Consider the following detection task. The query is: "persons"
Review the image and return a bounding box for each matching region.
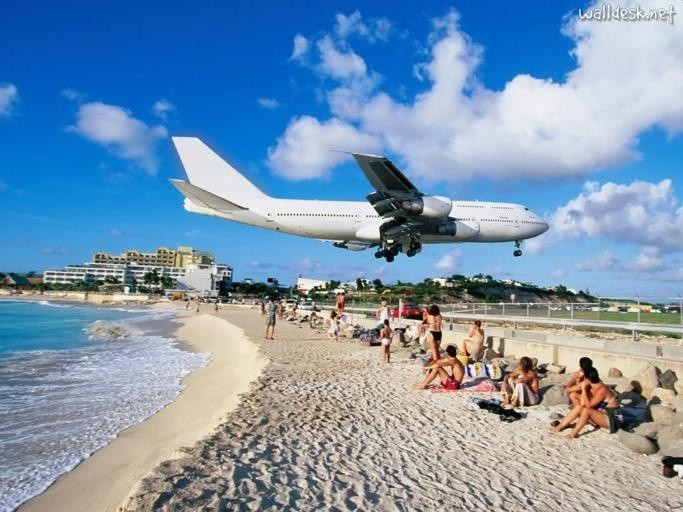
[276,302,283,319]
[563,356,593,408]
[287,307,296,317]
[263,296,276,339]
[375,297,388,325]
[299,314,309,322]
[409,344,465,391]
[259,300,265,315]
[378,318,392,363]
[498,356,540,408]
[420,304,443,361]
[460,319,482,337]
[326,310,340,339]
[550,366,626,439]
[310,312,317,326]
[335,291,344,313]
[333,292,340,304]
[458,327,484,357]
[415,307,432,339]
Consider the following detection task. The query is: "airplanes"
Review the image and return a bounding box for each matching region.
[171,135,549,264]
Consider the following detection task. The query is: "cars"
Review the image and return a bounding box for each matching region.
[280,298,316,310]
[392,303,423,319]
